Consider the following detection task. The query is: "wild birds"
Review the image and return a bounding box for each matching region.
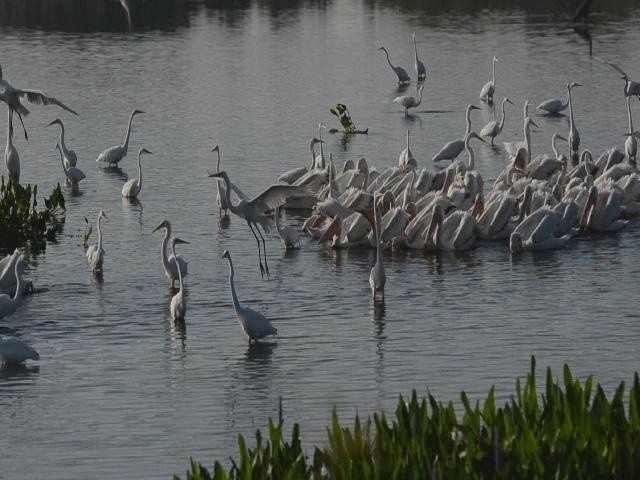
[4,104,154,196]
[375,32,430,85]
[394,86,429,118]
[149,218,192,320]
[0,247,41,365]
[619,71,640,99]
[210,98,640,298]
[474,54,502,109]
[536,76,583,151]
[85,210,108,272]
[219,250,278,346]
